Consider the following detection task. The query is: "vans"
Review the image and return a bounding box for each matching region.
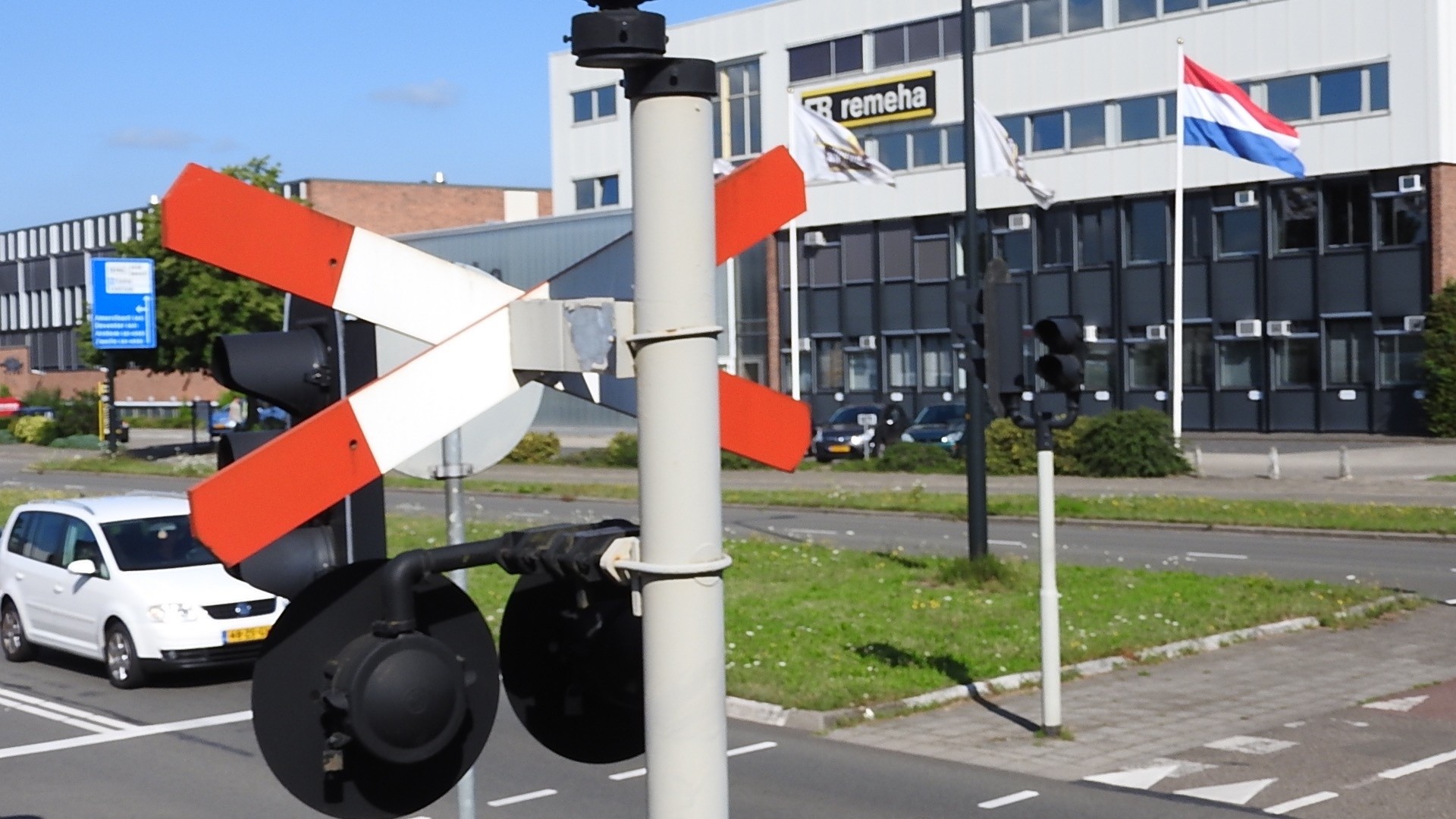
[0,487,291,690]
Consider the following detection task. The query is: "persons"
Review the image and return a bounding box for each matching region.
[228,395,242,424]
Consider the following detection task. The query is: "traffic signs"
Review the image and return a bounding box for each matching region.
[91,257,157,350]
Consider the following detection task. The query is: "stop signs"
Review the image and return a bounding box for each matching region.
[0,396,21,417]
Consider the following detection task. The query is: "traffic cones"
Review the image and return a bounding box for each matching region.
[1338,446,1352,480]
[1266,447,1280,479]
[1192,448,1205,477]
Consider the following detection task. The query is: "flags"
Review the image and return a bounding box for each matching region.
[1177,52,1306,180]
[972,98,1061,210]
[785,94,899,190]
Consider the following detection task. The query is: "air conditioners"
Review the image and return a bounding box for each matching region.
[1007,213,1031,231]
[1084,326,1099,341]
[1235,319,1262,340]
[804,232,826,249]
[1404,315,1426,332]
[859,335,876,350]
[1397,173,1421,194]
[1234,189,1254,206]
[799,337,811,352]
[1145,324,1167,341]
[1265,320,1291,335]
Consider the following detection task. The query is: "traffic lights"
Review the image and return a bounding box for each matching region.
[211,290,389,606]
[1033,314,1085,391]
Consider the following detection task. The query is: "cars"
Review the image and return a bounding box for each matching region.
[901,402,995,460]
[207,399,287,436]
[815,402,892,463]
